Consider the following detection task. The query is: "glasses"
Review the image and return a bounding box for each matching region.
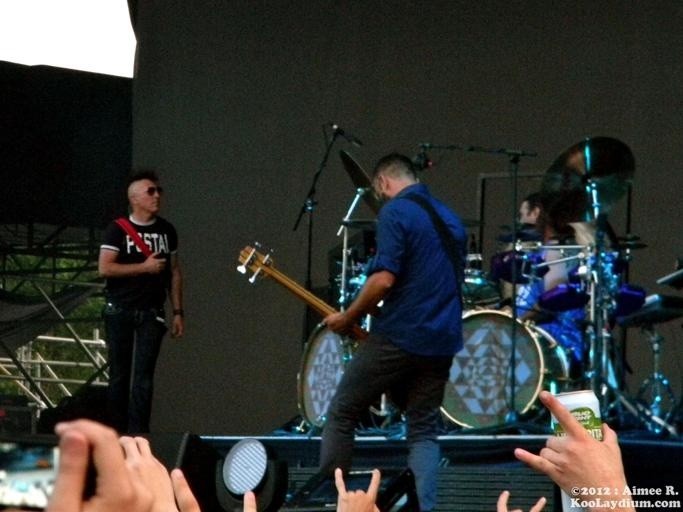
[136,186,164,198]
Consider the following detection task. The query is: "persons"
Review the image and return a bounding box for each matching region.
[99,178,184,435]
[502,192,578,320]
[315,152,468,509]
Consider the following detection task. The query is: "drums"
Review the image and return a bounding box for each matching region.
[460,252,501,306]
[296,321,365,430]
[540,135,636,223]
[439,309,566,429]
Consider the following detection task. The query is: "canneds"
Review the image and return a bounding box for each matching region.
[550,389,603,512]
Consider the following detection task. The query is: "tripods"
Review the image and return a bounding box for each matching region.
[514,180,683,442]
[278,190,406,436]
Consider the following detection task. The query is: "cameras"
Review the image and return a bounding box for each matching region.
[0,434,97,512]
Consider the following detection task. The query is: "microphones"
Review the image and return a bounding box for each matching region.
[328,119,362,147]
[411,154,438,172]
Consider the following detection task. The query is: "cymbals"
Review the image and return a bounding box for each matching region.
[497,223,539,243]
[340,149,383,214]
[489,251,548,284]
[337,218,375,230]
